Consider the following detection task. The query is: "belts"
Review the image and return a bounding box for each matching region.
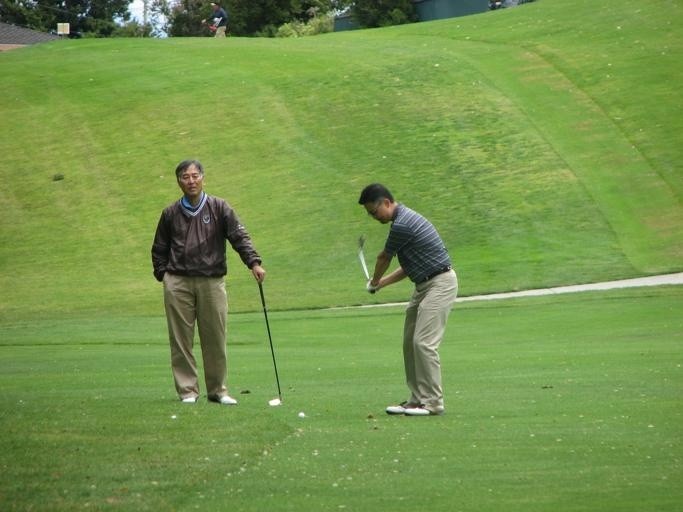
[417,265,451,284]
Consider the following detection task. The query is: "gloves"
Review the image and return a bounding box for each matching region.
[367,281,380,291]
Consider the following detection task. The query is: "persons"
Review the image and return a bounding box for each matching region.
[151,160,265,406]
[201,2,229,38]
[359,183,459,415]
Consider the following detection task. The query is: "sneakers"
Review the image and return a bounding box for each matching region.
[387,406,403,414]
[217,396,237,405]
[404,408,431,416]
[182,396,199,402]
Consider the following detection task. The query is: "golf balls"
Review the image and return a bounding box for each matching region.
[298,411,306,418]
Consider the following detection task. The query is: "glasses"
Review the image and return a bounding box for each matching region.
[367,201,382,216]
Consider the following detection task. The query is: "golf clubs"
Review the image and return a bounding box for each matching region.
[357,232,376,294]
[258,280,284,406]
